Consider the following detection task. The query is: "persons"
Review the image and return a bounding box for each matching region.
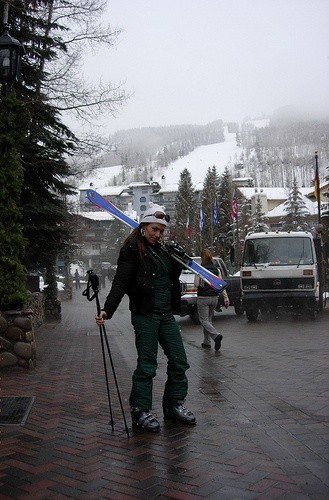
[194,249,230,351]
[94,205,198,431]
[74,265,118,289]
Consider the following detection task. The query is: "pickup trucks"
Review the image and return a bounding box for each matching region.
[178,254,245,324]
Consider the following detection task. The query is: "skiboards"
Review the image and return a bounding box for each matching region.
[87,188,228,292]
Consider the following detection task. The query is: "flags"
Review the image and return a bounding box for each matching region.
[169,189,237,240]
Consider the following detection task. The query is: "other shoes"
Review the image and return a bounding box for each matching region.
[202,342,211,348]
[214,335,223,350]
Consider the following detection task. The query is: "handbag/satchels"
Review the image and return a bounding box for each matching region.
[218,268,229,309]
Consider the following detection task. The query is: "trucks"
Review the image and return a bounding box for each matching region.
[240,228,329,323]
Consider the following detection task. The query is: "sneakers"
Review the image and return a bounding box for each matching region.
[130,405,160,432]
[163,405,196,425]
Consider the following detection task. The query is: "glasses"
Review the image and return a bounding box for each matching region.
[144,211,170,222]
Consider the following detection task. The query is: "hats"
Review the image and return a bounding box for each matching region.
[141,202,168,226]
